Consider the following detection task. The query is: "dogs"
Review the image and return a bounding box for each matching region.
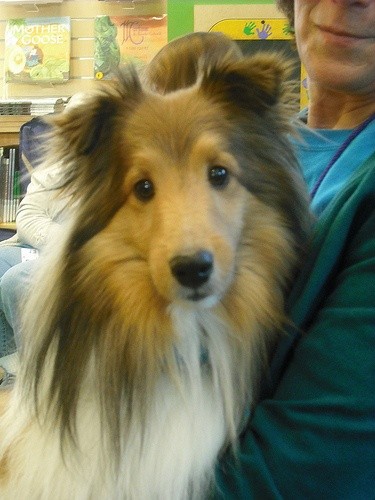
[0,38,311,500]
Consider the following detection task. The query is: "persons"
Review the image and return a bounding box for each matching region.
[204,0,375,500]
[0,154,73,396]
